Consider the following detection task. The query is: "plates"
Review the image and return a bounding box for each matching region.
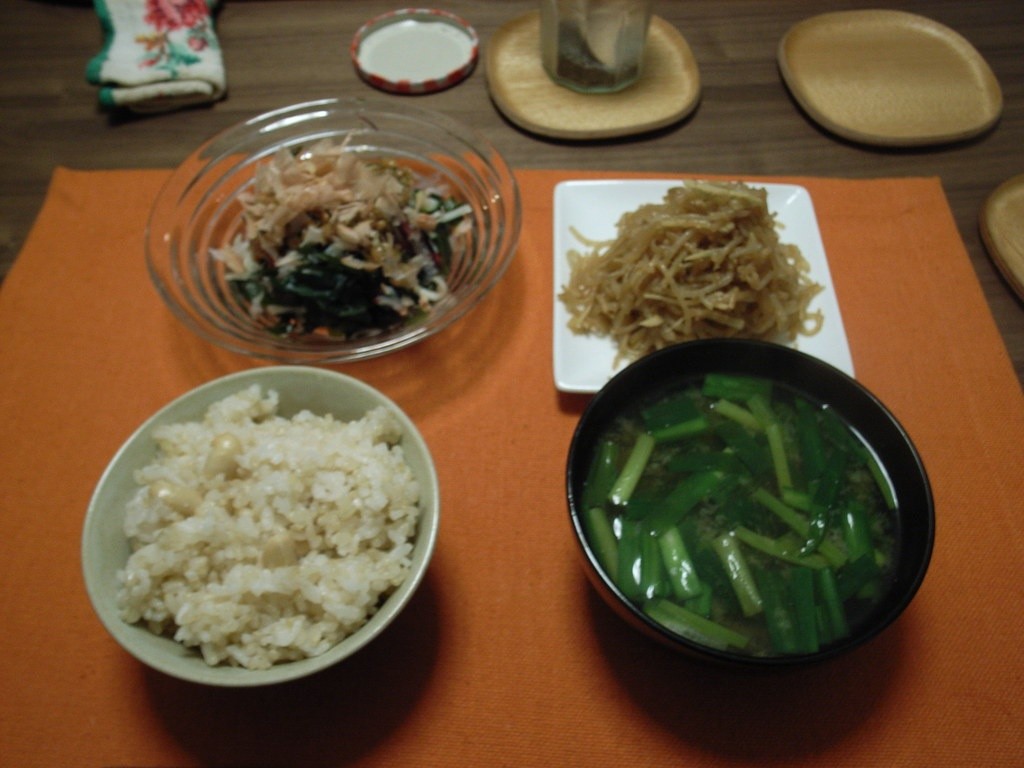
[551,179,856,393]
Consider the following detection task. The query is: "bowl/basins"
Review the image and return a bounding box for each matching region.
[566,338,936,675]
[143,95,522,362]
[81,365,439,686]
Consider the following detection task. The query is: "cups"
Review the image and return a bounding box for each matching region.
[536,0,653,95]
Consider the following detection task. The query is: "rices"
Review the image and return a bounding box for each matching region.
[112,386,421,670]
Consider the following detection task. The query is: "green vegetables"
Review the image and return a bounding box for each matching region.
[221,187,471,341]
[582,377,902,655]
